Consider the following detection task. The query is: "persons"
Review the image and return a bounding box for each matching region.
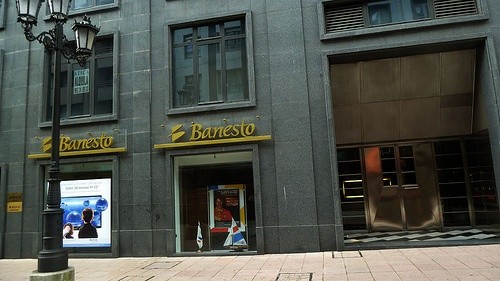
[214,193,234,222]
[63,208,98,238]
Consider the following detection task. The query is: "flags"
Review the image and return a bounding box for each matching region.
[196,220,204,250]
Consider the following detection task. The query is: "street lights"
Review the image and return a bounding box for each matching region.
[13,0,103,281]
